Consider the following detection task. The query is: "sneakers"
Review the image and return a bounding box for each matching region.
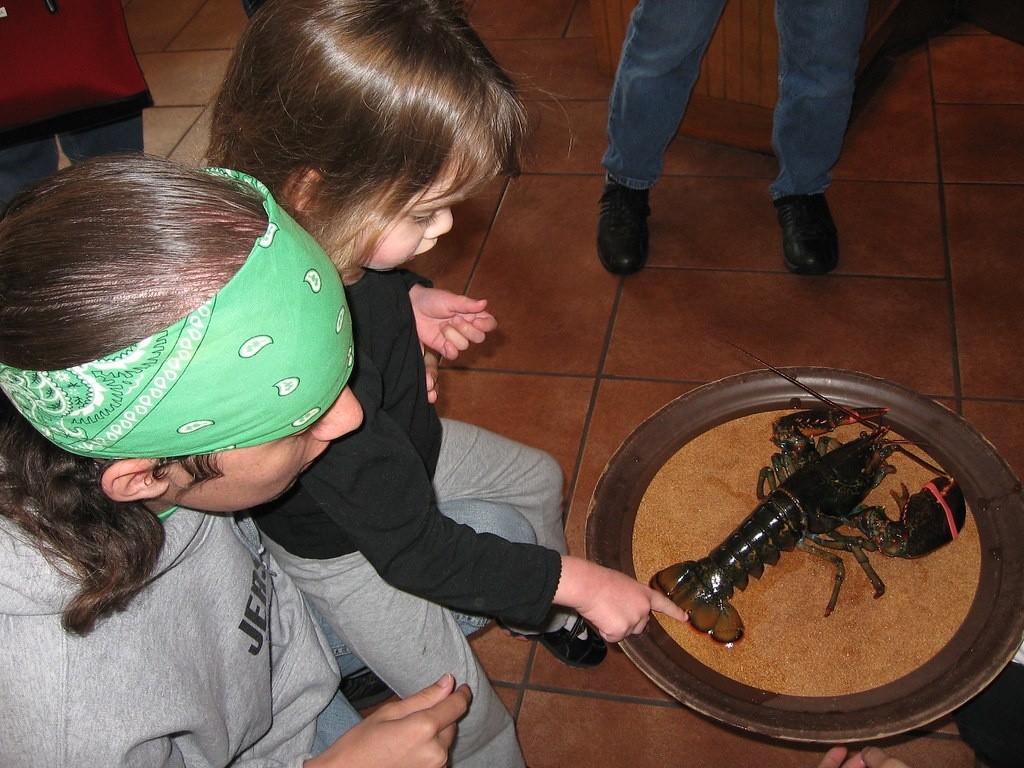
[773,194,840,273]
[596,171,652,275]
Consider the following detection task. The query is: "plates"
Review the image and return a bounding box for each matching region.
[586,366,1024,743]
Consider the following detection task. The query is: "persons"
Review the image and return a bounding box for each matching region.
[0,0,689,768]
[595,0,867,276]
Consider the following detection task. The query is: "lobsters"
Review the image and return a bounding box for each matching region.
[648,339,966,647]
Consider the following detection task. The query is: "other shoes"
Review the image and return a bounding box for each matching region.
[338,670,395,710]
[495,612,608,670]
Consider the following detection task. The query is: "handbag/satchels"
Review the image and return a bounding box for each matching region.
[0,0,152,150]
